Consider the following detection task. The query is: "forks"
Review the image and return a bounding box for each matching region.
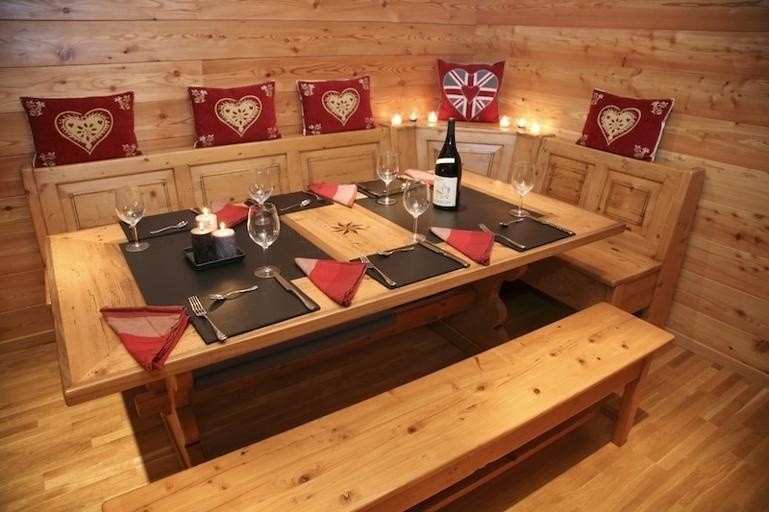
[187,293,228,344]
[478,222,528,250]
[358,252,398,288]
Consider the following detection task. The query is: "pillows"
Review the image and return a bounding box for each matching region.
[436,56,505,127]
[575,87,675,163]
[188,81,282,149]
[295,74,376,136]
[19,90,143,168]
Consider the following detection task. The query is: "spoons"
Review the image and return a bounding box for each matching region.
[150,220,189,234]
[378,245,417,257]
[207,284,260,301]
[280,198,312,212]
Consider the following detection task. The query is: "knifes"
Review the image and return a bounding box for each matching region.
[420,240,465,266]
[270,270,318,312]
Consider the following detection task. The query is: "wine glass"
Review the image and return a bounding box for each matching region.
[376,151,400,207]
[246,202,281,280]
[508,159,536,220]
[403,177,430,245]
[246,166,275,227]
[114,186,150,254]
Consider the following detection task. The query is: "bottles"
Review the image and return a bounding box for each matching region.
[432,117,463,212]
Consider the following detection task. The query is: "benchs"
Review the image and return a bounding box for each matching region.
[101,298,676,512]
